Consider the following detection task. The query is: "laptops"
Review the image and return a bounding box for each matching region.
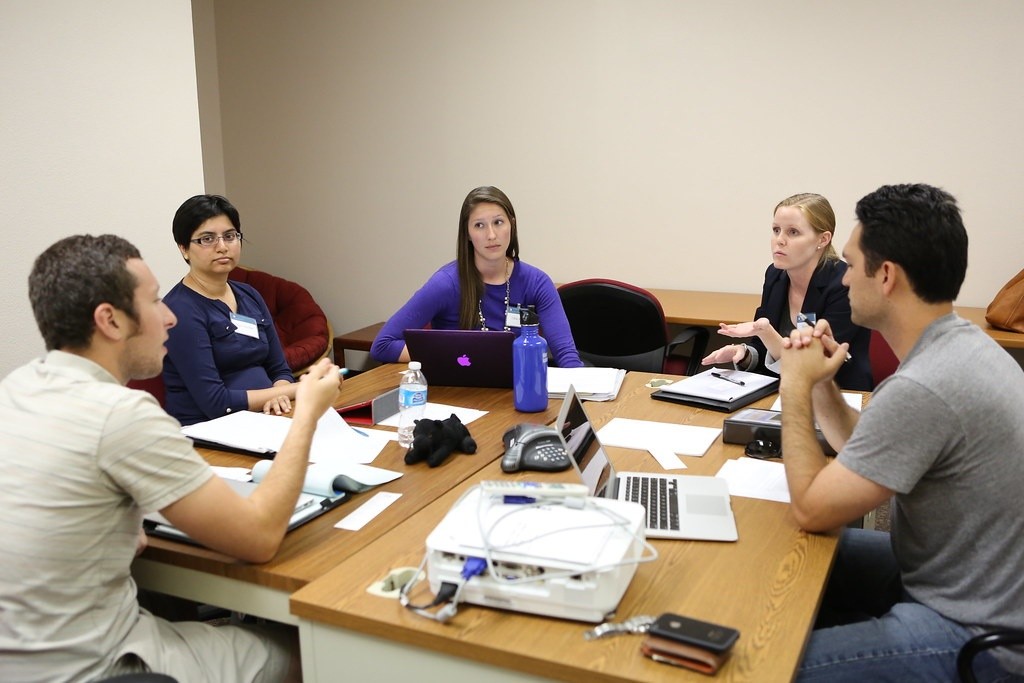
[402,329,517,389]
[555,383,739,542]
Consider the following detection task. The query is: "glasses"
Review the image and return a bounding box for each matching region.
[190,232,241,246]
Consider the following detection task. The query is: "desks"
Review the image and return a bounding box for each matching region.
[129,361,880,683]
[332,322,388,379]
[641,288,1024,351]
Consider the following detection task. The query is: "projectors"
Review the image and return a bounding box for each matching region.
[425,479,647,623]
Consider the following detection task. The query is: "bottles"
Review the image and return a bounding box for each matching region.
[513,308,548,412]
[398,361,429,450]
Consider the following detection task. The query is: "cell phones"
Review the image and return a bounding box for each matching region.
[649,613,740,652]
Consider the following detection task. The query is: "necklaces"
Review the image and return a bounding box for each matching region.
[478,256,511,332]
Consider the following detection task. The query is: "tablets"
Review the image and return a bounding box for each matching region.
[334,385,400,427]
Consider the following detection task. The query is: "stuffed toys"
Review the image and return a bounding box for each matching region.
[404,413,477,469]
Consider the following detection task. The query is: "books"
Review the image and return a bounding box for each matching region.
[155,459,403,540]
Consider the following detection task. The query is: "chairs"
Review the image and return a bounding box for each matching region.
[538,278,710,377]
[227,264,333,378]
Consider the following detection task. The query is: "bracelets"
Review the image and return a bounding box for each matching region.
[741,342,747,352]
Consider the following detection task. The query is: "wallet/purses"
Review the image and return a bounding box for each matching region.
[640,635,731,675]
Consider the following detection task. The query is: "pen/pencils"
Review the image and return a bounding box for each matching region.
[352,426,369,438]
[339,367,349,375]
[711,372,745,386]
[797,311,852,363]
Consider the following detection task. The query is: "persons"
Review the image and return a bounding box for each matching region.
[701,192,876,392]
[370,185,584,369]
[779,181,1024,683]
[161,195,301,427]
[1,233,343,683]
